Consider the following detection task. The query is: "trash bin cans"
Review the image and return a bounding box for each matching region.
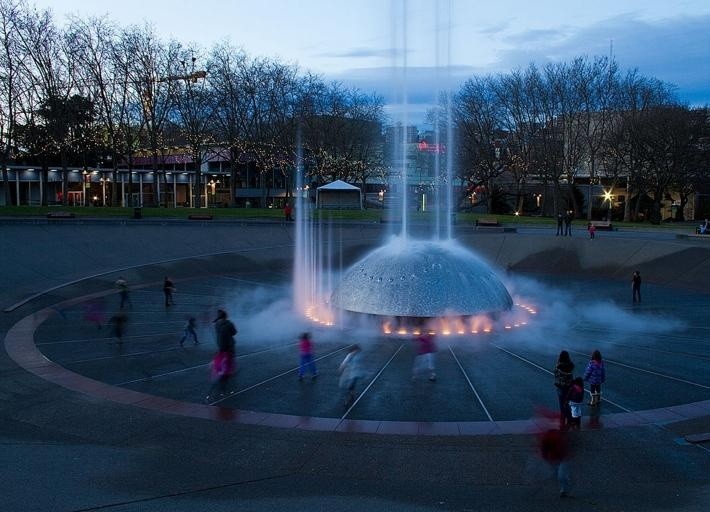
[451,214,456,225]
[134,207,141,219]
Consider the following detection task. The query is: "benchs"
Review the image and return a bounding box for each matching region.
[380,214,403,225]
[47,210,75,218]
[474,217,498,226]
[590,219,611,231]
[186,212,213,220]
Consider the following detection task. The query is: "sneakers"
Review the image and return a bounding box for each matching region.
[430,375,436,381]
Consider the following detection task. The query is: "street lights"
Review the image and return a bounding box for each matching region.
[416,167,423,184]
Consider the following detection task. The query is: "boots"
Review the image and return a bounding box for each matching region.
[587,391,600,405]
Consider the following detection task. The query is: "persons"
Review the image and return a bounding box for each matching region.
[163,276,176,307]
[556,210,563,236]
[632,270,641,303]
[701,219,710,234]
[339,344,362,405]
[554,350,585,429]
[534,430,575,494]
[414,328,438,380]
[298,333,319,380]
[119,282,132,308]
[584,350,603,405]
[589,224,596,239]
[109,312,127,338]
[179,318,201,347]
[213,310,237,396]
[565,210,572,236]
[285,203,293,221]
